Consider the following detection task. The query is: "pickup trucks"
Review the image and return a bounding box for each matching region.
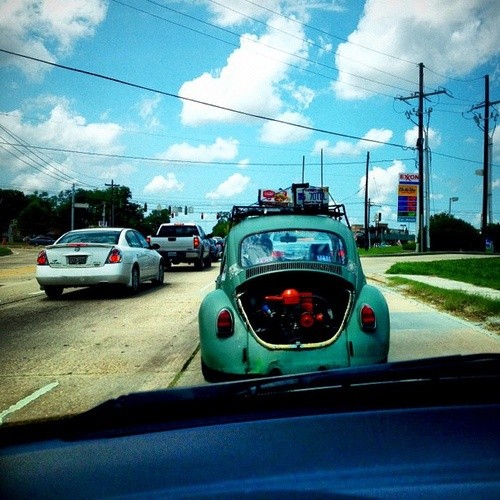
[145,222,212,271]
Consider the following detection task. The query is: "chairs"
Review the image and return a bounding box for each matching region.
[302,241,335,265]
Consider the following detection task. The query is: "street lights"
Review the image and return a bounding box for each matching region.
[448,197,458,217]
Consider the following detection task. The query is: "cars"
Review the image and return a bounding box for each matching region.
[29,235,55,246]
[214,236,226,259]
[34,227,166,298]
[207,238,220,262]
[197,215,392,383]
